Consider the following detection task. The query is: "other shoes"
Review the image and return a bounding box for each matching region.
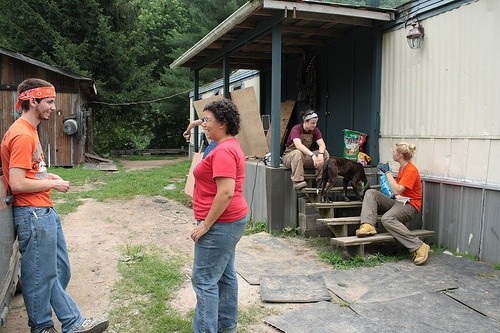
[318,179,330,189]
[356,222,377,238]
[294,179,308,190]
[411,241,430,265]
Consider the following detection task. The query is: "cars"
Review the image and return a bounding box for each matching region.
[0,146,25,332]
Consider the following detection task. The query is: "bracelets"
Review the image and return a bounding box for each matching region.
[318,151,324,155]
[201,220,210,230]
[311,153,314,157]
[385,171,389,174]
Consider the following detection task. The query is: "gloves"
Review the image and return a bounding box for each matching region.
[378,161,390,174]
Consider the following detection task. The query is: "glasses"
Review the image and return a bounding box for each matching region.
[203,118,215,122]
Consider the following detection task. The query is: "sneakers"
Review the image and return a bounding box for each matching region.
[65,319,109,332]
[34,326,59,333]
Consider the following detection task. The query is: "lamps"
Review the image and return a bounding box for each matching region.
[404,14,424,49]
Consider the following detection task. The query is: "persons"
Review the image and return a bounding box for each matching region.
[0,78,109,333]
[356,142,430,264]
[282,108,329,190]
[183,99,248,333]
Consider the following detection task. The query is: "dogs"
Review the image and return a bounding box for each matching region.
[314,155,370,205]
